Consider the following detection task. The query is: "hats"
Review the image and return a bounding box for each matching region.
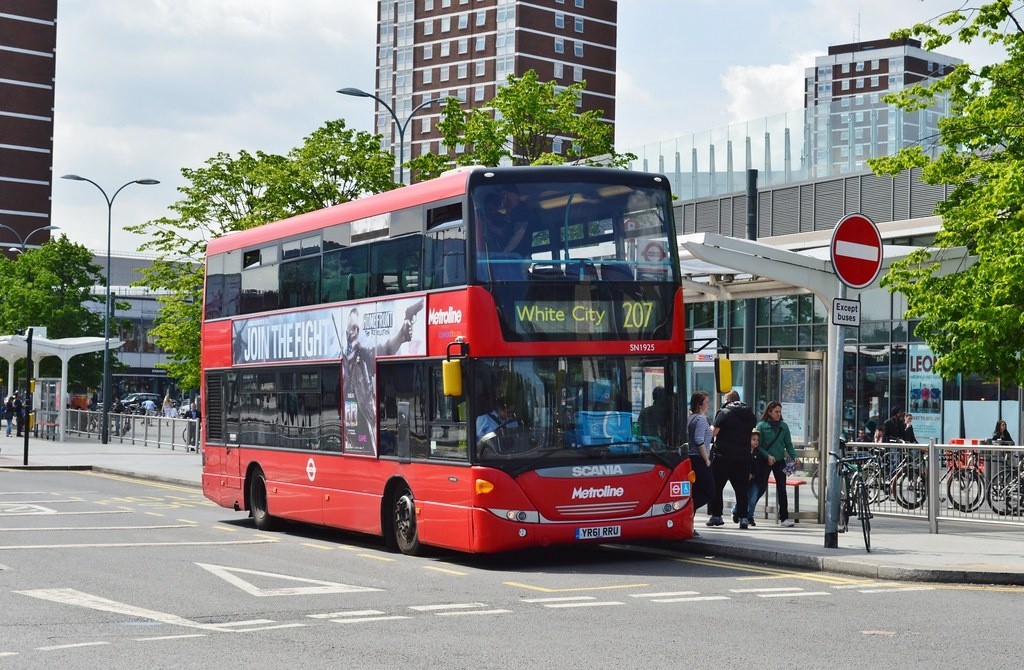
[752,428,760,433]
[891,405,901,416]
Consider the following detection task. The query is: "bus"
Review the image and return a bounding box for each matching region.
[200,168,732,559]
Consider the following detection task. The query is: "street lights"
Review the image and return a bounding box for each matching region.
[61,174,162,448]
[337,88,467,187]
[0,224,62,258]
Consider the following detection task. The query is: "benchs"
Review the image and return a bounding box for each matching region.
[765,477,807,523]
[35,422,60,441]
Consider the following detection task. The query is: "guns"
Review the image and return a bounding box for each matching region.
[403,298,424,342]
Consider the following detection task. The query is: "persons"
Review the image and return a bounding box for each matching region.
[686,391,714,538]
[706,390,758,530]
[992,420,1016,446]
[161,394,178,427]
[635,386,680,451]
[4,395,14,437]
[182,402,201,452]
[338,308,413,456]
[475,396,520,451]
[13,389,25,437]
[112,394,125,436]
[141,396,157,426]
[92,389,98,411]
[481,182,535,277]
[856,404,928,500]
[732,401,801,527]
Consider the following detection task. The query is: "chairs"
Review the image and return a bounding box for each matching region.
[443,251,528,285]
[566,258,599,282]
[600,258,634,281]
[532,263,565,281]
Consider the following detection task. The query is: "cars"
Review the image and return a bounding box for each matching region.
[113,393,160,416]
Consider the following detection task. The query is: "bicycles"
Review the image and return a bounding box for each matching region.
[829,450,879,552]
[810,444,1024,507]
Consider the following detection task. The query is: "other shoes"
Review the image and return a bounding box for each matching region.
[781,520,794,527]
[693,528,702,538]
[6,434,12,437]
[748,517,756,527]
[16,433,23,437]
[732,508,739,523]
[706,517,724,526]
[739,517,748,529]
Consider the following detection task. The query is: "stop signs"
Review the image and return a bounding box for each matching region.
[828,212,883,292]
